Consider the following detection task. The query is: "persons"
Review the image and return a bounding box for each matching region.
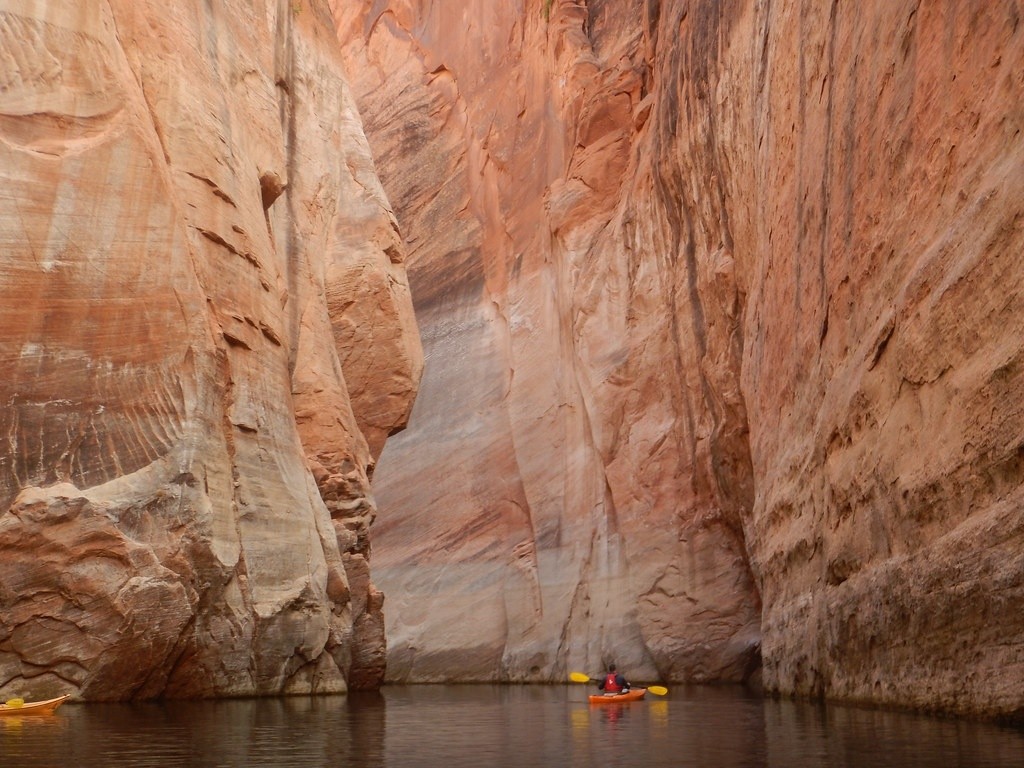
[596,664,630,696]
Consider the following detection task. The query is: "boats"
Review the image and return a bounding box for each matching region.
[0,693,72,716]
[588,688,647,704]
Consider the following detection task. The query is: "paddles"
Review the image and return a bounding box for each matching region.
[0,698,24,709]
[569,672,668,696]
